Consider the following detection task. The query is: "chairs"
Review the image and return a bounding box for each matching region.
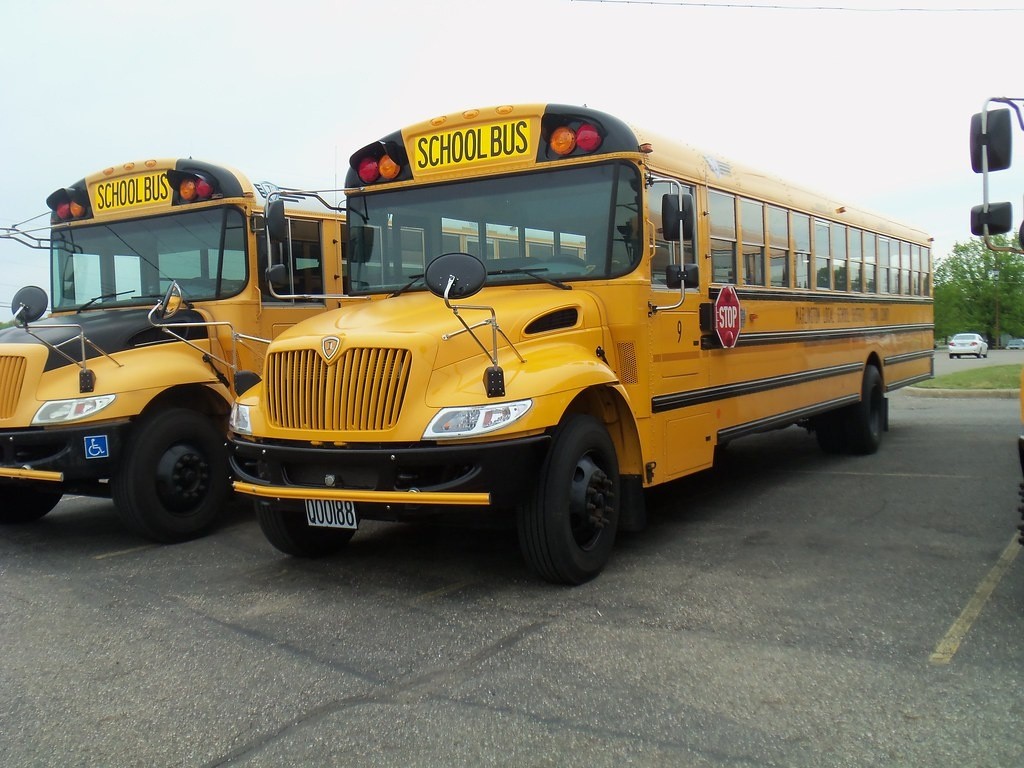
[291,266,323,303]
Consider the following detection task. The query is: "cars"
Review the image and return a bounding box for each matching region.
[1006,339,1024,350]
[949,334,989,359]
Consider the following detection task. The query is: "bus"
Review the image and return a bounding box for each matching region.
[646,211,797,290]
[148,99,935,586]
[1,157,587,545]
[970,95,1024,553]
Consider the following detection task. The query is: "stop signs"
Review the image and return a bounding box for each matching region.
[714,285,742,349]
